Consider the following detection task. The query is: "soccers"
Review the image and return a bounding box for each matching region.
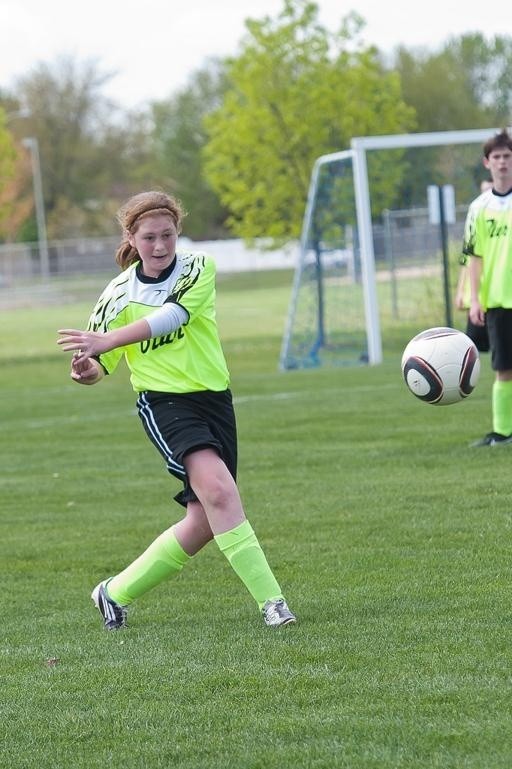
[400,327,481,405]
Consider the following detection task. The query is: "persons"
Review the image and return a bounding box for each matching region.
[455,176,495,355]
[54,190,295,632]
[463,130,512,446]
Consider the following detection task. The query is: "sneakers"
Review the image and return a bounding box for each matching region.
[91,577,129,632]
[261,596,297,627]
[470,432,511,449]
